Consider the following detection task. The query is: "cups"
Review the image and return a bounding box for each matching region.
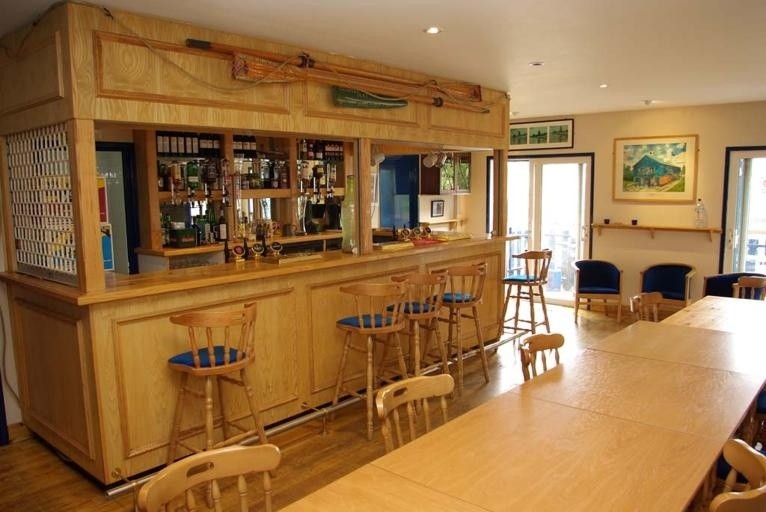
[632,219,637,225]
[604,219,611,224]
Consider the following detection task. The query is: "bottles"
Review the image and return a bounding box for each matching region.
[217,210,226,239]
[694,199,704,227]
[157,131,256,159]
[156,158,218,191]
[302,139,344,161]
[241,159,289,189]
[340,175,356,253]
[191,216,200,246]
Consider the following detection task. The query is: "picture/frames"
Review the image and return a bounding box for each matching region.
[613,133,699,205]
[508,118,573,151]
[431,200,444,217]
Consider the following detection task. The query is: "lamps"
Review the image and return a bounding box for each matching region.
[423,155,438,168]
[435,151,447,168]
[371,151,385,166]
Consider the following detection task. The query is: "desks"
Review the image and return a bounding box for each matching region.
[279,296,766,512]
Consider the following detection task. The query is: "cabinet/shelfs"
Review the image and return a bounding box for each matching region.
[133,127,347,249]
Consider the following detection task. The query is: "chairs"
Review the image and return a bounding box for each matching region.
[520,334,564,381]
[697,389,766,512]
[166,301,276,507]
[377,270,454,401]
[573,260,766,324]
[329,276,417,440]
[495,250,553,342]
[375,373,455,452]
[421,262,489,397]
[137,444,281,512]
